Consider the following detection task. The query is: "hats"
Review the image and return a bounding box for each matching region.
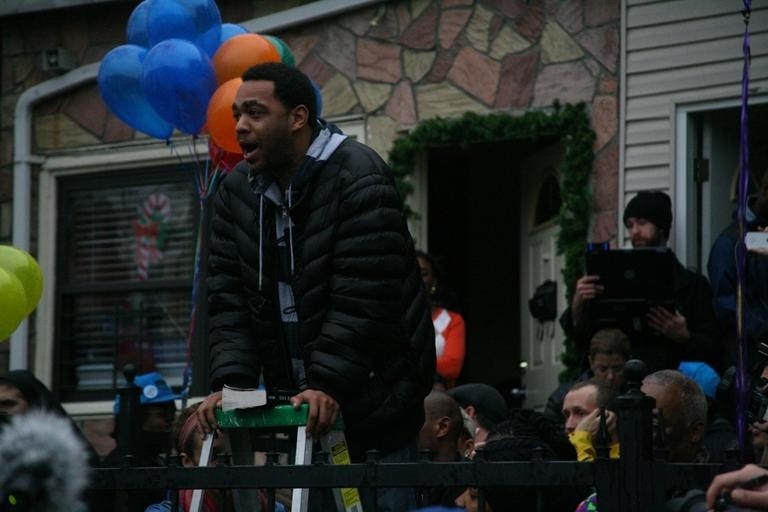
[678,361,720,400]
[623,190,672,234]
[447,383,507,427]
[113,373,190,415]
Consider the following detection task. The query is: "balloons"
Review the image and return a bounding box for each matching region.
[99,0,323,174]
[0,243,45,342]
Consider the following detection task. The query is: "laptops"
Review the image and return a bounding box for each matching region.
[584,248,677,315]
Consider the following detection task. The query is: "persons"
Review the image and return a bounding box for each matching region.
[558,188,713,357]
[0,327,766,512]
[706,143,768,368]
[195,62,437,512]
[413,246,466,388]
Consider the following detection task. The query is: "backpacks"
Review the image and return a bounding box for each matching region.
[528,279,556,324]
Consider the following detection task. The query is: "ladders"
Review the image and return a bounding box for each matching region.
[187,402,364,512]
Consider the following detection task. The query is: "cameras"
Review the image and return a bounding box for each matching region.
[668,488,756,511]
